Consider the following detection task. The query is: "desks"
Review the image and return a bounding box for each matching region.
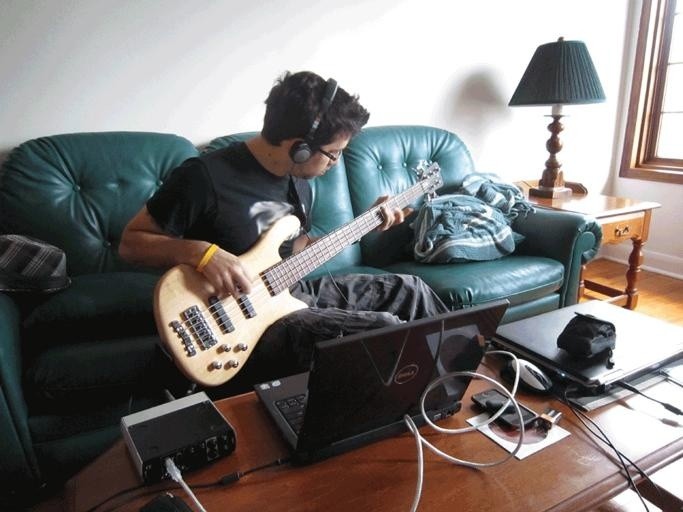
[67,300,682,512]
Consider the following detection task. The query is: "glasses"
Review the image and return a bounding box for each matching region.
[315,145,342,164]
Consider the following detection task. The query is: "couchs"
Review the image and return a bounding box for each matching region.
[1,126,606,512]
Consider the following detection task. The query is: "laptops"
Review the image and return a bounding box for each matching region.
[254,298,509,464]
[493,299,683,396]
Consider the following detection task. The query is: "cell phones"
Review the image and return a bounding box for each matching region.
[471,389,538,430]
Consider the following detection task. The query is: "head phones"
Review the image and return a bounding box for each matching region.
[288,77,338,164]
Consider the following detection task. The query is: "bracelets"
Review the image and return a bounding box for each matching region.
[196,244,219,272]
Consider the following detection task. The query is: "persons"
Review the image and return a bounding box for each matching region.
[118,70,452,371]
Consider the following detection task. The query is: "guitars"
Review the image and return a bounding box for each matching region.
[153,160,444,386]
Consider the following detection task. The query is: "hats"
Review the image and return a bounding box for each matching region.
[2,233,72,294]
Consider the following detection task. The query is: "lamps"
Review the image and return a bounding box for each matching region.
[508,35,607,201]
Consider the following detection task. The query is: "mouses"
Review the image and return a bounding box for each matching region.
[501,355,552,394]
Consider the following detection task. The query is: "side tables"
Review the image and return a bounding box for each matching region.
[524,193,662,310]
